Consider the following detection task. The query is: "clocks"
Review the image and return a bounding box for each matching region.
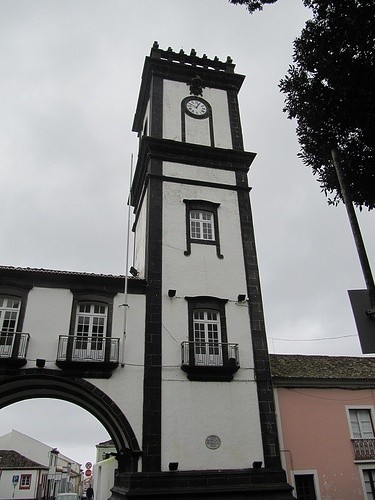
[181,96,213,119]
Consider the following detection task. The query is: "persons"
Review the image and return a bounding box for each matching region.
[85,483,94,500]
